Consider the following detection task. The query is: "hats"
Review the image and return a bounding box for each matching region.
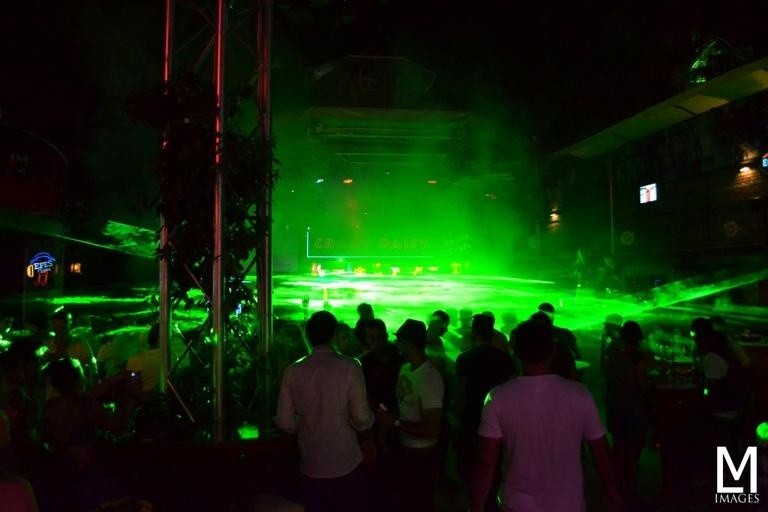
[394,319,430,345]
